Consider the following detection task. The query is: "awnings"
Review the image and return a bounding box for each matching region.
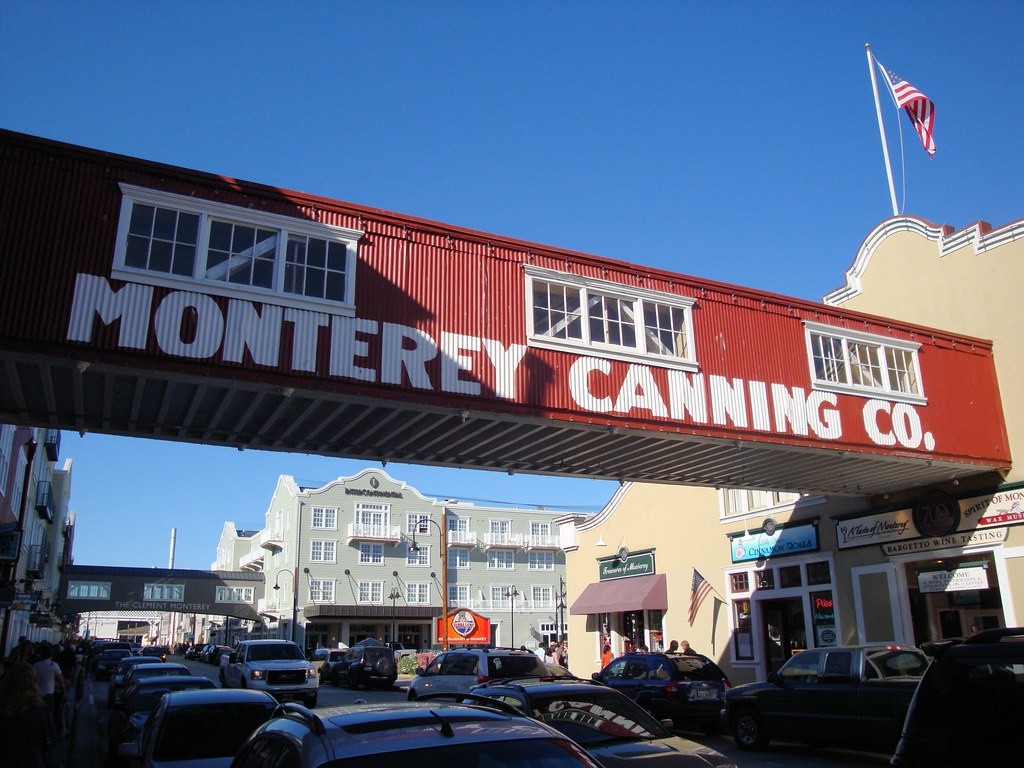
[569,573,668,615]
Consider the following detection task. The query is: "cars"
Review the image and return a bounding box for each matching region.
[90,641,287,768]
[185,643,234,666]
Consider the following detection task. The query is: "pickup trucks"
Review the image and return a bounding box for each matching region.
[219,639,319,709]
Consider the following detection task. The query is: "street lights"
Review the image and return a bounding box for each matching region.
[408,515,449,650]
[505,585,520,651]
[273,570,296,640]
[388,587,402,650]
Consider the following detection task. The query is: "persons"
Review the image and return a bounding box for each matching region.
[0,636,92,768]
[520,639,568,675]
[601,641,696,681]
[166,642,190,655]
[970,626,983,643]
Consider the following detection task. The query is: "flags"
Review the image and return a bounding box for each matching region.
[876,63,936,160]
[687,569,713,627]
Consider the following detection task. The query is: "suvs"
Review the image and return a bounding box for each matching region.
[407,645,553,703]
[591,626,1024,768]
[309,645,398,690]
[462,675,737,768]
[227,691,605,768]
